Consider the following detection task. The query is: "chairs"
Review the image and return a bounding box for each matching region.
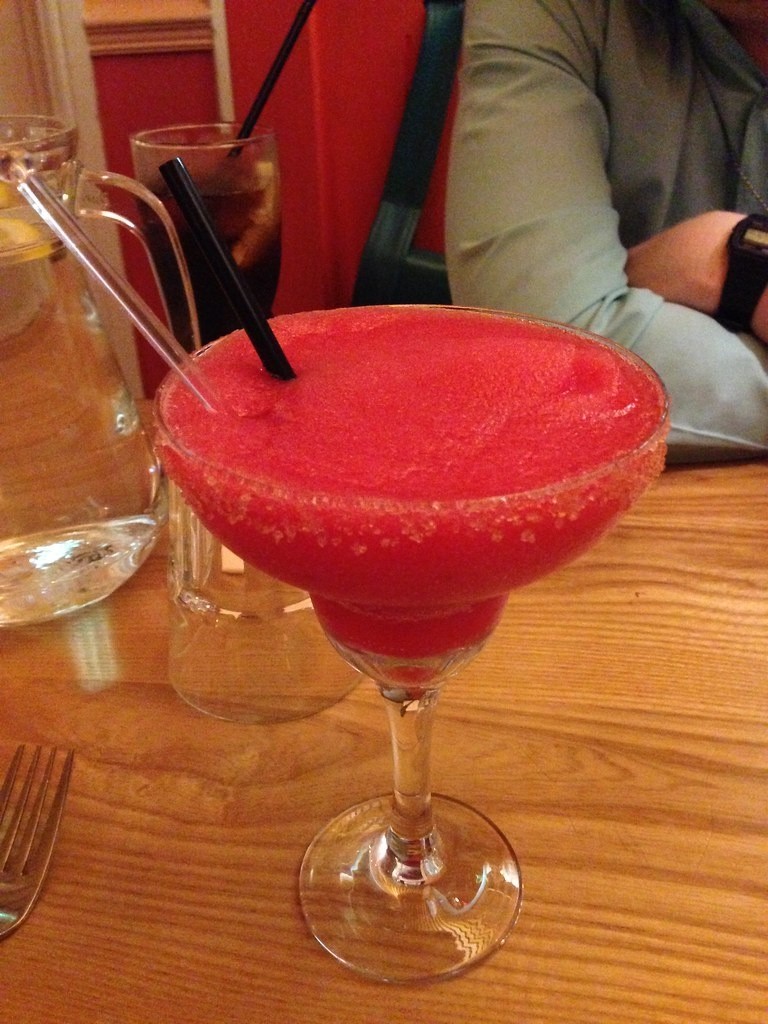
[350,0,464,307]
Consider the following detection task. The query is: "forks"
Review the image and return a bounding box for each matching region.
[0,743,76,936]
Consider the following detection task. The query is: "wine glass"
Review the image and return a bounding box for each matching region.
[146,301,673,988]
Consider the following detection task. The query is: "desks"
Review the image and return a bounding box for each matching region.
[0,400,767,1024]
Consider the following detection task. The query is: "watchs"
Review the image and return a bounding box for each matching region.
[710,213,768,331]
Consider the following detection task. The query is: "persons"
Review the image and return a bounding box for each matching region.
[445,0,768,464]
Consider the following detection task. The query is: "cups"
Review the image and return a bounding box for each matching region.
[132,125,282,354]
[163,471,367,724]
[0,113,204,628]
